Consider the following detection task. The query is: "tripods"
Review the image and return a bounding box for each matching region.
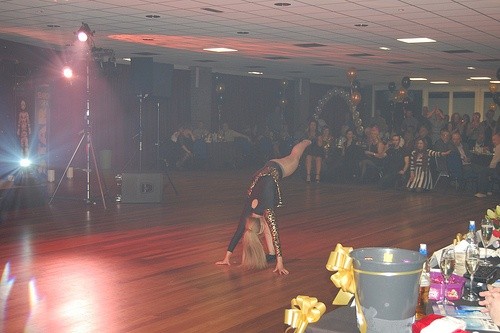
[148,97,178,194]
[47,49,108,209]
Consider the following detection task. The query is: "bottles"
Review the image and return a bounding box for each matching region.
[416,243,430,319]
[465,220,480,246]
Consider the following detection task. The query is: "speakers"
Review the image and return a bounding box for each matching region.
[129,56,152,87]
[148,62,176,101]
[121,172,163,203]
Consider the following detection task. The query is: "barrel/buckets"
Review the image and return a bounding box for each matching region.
[349,246,428,333]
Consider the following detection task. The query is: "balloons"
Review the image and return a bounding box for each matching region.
[215,74,225,103]
[312,68,364,136]
[388,76,410,107]
[278,79,289,107]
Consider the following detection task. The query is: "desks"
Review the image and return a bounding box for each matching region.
[306,228,500,332]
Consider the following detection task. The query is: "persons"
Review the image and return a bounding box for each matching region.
[478,284,500,327]
[167,107,500,196]
[216,139,313,275]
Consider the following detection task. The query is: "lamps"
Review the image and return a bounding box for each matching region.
[78,22,90,42]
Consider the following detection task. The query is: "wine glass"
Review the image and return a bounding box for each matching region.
[462,244,481,301]
[480,219,494,266]
[436,248,456,306]
